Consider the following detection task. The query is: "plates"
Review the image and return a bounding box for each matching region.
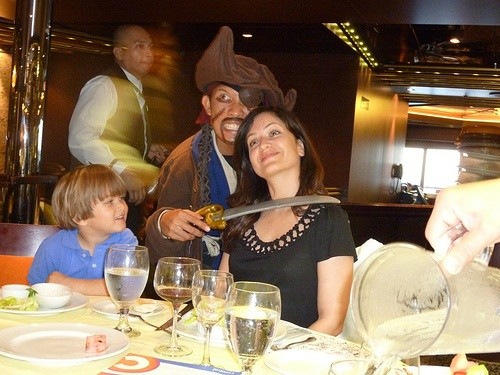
[199,322,287,341]
[0,291,89,314]
[264,348,346,375]
[90,298,165,316]
[0,321,130,364]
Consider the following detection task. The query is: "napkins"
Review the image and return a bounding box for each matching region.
[265,329,340,372]
[172,320,225,347]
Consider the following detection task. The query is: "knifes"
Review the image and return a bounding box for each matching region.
[155,302,194,331]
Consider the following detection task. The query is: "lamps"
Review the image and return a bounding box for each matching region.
[447,25,465,45]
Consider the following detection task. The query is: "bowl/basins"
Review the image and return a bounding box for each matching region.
[32,283,70,309]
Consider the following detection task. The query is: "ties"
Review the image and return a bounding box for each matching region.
[139,91,152,161]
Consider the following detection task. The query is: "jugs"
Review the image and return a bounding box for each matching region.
[350,223,500,360]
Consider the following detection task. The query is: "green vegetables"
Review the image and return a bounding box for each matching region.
[0,288,40,311]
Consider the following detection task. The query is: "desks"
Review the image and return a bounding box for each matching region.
[0,295,366,375]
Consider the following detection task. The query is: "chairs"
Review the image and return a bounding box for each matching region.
[0,222,61,286]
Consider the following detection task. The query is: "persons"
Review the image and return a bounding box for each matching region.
[28,164,138,295]
[214,104,359,336]
[68,25,162,229]
[425,177,500,275]
[142,26,297,301]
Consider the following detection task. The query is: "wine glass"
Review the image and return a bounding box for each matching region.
[104,244,149,339]
[192,268,234,372]
[153,257,205,357]
[223,281,281,375]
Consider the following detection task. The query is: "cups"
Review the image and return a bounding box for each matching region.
[0,284,31,298]
[328,359,415,375]
